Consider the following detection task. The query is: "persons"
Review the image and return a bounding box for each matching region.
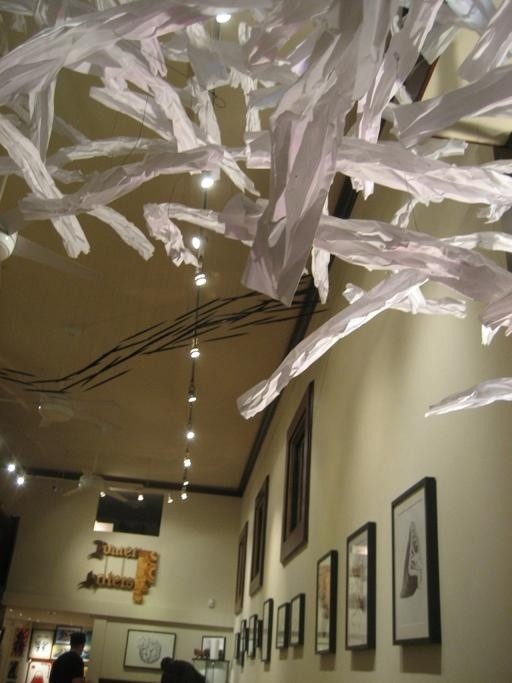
[161,657,206,683]
[49,632,87,683]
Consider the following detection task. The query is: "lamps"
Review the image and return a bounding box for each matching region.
[199,170,214,190]
[186,390,198,403]
[183,450,193,468]
[193,266,207,287]
[189,338,201,358]
[185,423,196,440]
[6,462,26,487]
[98,470,195,505]
[192,235,208,250]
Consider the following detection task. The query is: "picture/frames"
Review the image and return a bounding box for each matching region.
[390,475,441,648]
[313,549,337,656]
[233,593,307,667]
[122,627,177,671]
[345,521,377,649]
[23,623,92,683]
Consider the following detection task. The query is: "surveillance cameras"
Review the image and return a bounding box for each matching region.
[52,485,57,492]
